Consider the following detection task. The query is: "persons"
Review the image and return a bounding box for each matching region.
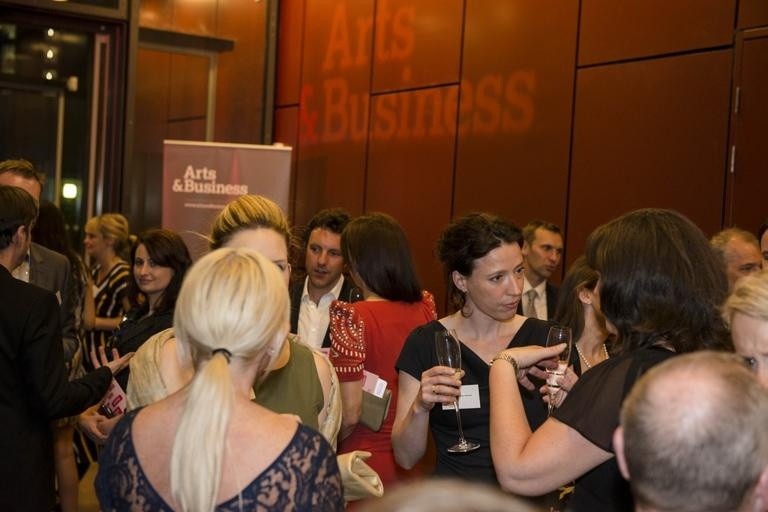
[2,157,768,512]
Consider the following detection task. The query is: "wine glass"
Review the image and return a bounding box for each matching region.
[545,323,573,418]
[434,328,480,452]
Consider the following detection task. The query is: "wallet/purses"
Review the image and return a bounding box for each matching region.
[360,390,391,432]
[337,451,383,502]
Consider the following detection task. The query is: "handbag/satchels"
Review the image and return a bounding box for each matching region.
[79,462,100,512]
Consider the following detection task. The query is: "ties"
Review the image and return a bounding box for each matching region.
[527,290,537,319]
